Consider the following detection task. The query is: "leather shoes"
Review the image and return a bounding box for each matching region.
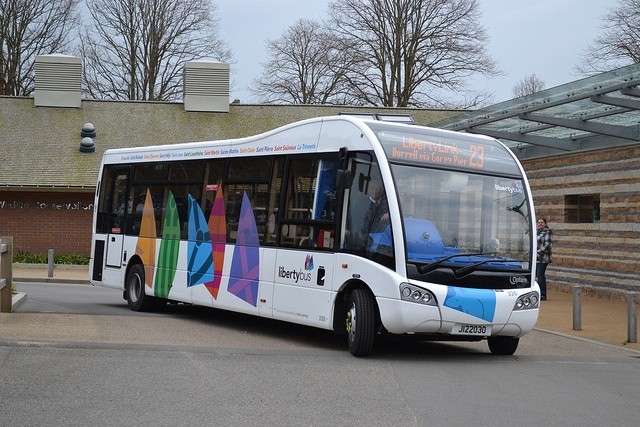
[541,294,547,301]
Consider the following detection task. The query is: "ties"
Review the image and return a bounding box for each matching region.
[375,200,379,208]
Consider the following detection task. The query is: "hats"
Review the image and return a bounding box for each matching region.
[139,190,147,196]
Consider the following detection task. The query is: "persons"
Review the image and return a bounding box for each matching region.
[345,183,389,252]
[536,218,552,300]
[122,187,134,213]
[136,191,147,213]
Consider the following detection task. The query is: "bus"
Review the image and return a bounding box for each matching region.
[87,114,542,356]
